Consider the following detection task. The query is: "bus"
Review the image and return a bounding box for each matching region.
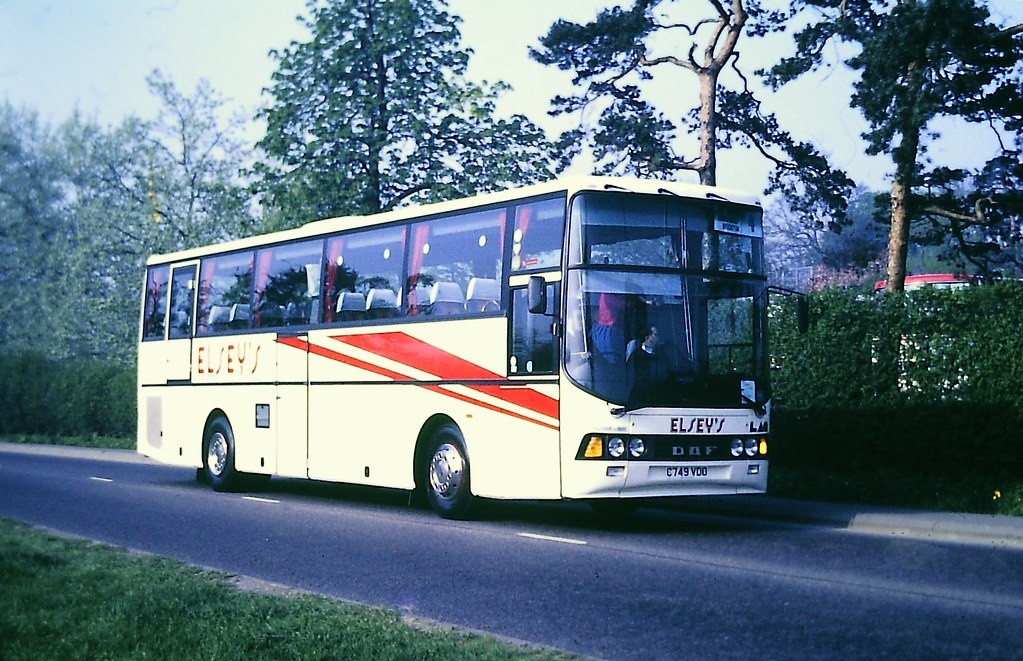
[134,175,810,518]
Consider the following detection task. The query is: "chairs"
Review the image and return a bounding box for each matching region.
[185,279,498,336]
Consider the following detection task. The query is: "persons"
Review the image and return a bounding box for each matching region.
[567,277,637,367]
[627,323,672,382]
